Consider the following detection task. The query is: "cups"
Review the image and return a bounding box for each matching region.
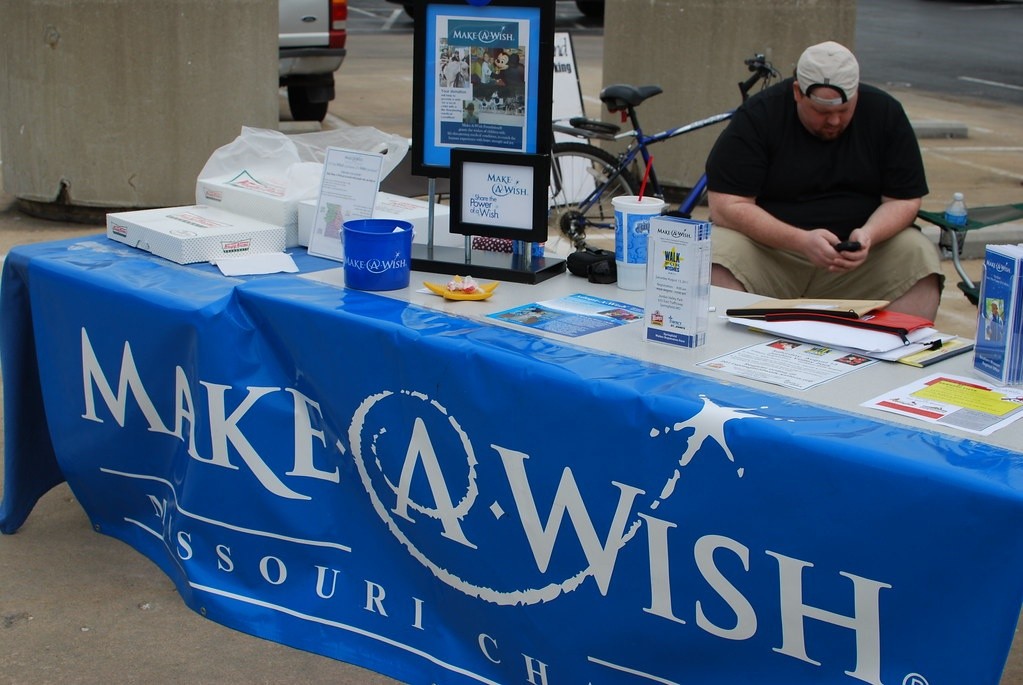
[611,196,666,291]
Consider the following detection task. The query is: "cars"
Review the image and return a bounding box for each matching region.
[278,0,348,123]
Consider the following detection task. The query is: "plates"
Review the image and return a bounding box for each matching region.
[422,275,500,301]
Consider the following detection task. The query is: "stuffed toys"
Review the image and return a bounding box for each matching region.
[491,49,526,99]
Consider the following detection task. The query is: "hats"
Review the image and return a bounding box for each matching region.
[505,53,520,65]
[465,103,474,110]
[797,41,859,105]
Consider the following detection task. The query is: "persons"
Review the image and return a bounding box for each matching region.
[440,46,470,86]
[705,41,946,322]
[479,51,525,107]
[462,102,479,125]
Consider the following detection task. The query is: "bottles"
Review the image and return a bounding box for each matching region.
[938,192,968,260]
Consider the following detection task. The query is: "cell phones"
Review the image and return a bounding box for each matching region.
[834,241,861,252]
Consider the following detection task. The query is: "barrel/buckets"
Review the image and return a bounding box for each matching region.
[339,218,417,291]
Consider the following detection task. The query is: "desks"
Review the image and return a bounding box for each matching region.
[1,235,1023,685]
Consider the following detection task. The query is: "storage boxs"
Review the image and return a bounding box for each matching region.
[107,204,288,266]
[284,224,298,248]
[297,189,481,247]
[643,234,713,350]
[971,263,1023,387]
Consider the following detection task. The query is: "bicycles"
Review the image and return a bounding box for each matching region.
[544,52,782,258]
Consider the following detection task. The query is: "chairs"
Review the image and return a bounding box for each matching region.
[918,203,1023,307]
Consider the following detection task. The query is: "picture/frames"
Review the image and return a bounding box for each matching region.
[449,147,551,242]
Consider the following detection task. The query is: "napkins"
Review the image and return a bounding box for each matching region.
[209,252,300,276]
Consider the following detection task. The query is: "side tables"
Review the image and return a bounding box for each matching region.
[197,168,319,224]
